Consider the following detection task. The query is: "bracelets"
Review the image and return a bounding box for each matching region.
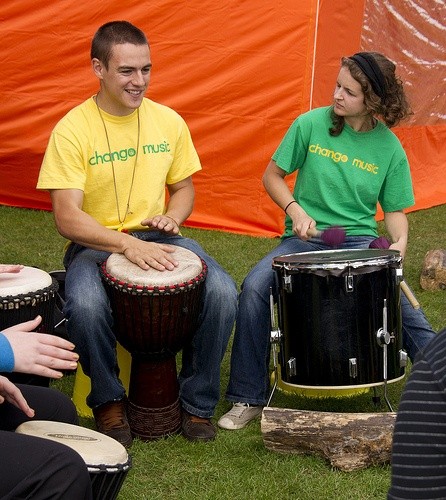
[284,201,297,214]
[167,216,179,226]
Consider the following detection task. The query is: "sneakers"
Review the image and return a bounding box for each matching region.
[181,411,216,442]
[93,401,132,447]
[219,402,264,430]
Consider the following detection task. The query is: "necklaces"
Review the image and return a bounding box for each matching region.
[96,91,140,233]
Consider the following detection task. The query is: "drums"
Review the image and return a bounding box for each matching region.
[270,248,408,390]
[14,420,133,500]
[98,243,209,442]
[0,264,60,389]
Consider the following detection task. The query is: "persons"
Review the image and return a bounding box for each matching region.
[387,325,446,500]
[37,21,236,449]
[0,375,90,500]
[0,263,80,379]
[218,51,436,430]
